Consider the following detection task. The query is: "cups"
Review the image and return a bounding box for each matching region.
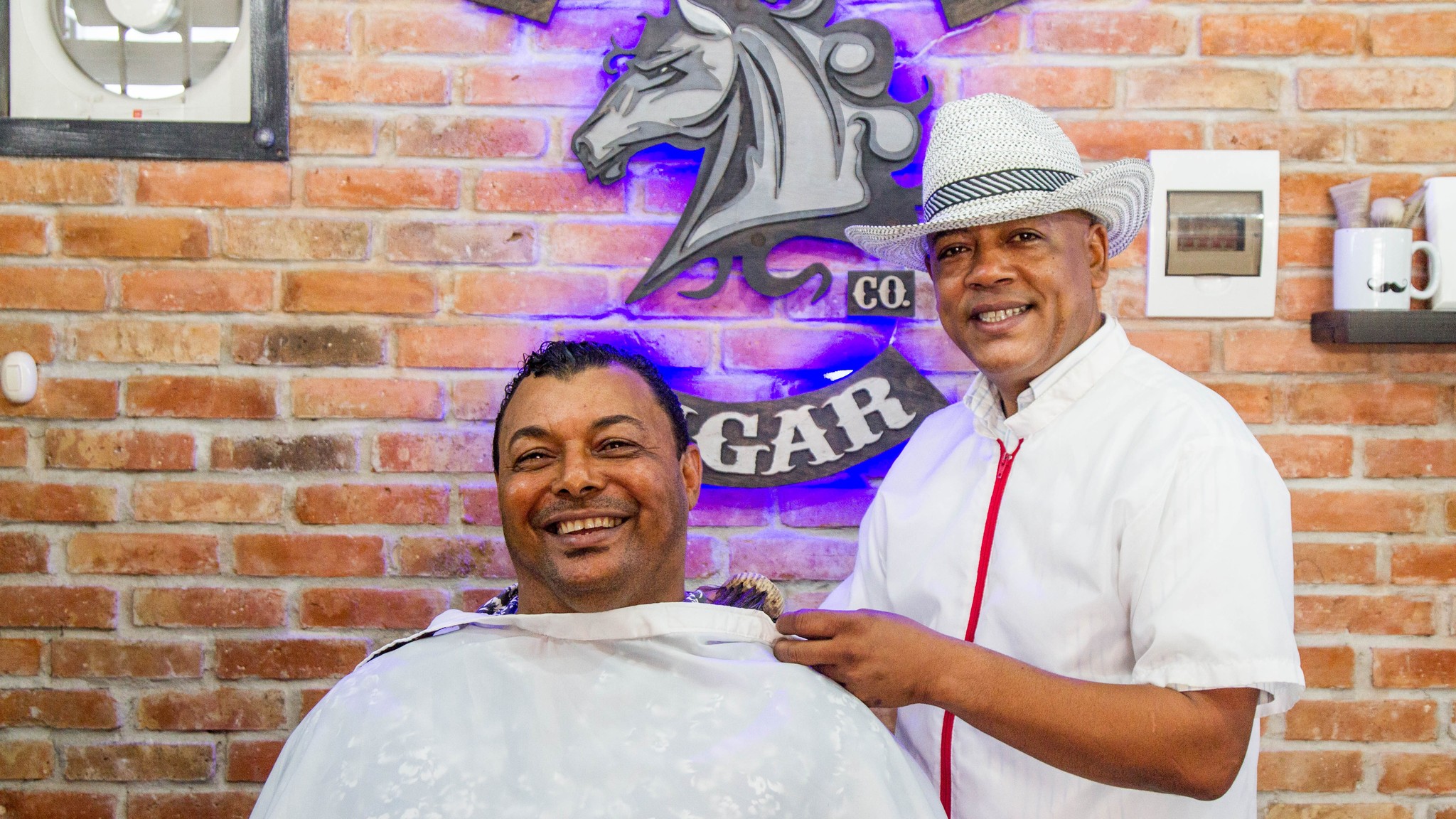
[1424,176,1456,310]
[1332,227,1440,310]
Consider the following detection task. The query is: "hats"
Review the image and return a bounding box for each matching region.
[844,93,1154,273]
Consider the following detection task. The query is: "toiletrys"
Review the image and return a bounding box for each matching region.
[1330,175,1372,229]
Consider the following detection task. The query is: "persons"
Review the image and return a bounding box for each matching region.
[772,93,1305,819]
[247,339,950,819]
